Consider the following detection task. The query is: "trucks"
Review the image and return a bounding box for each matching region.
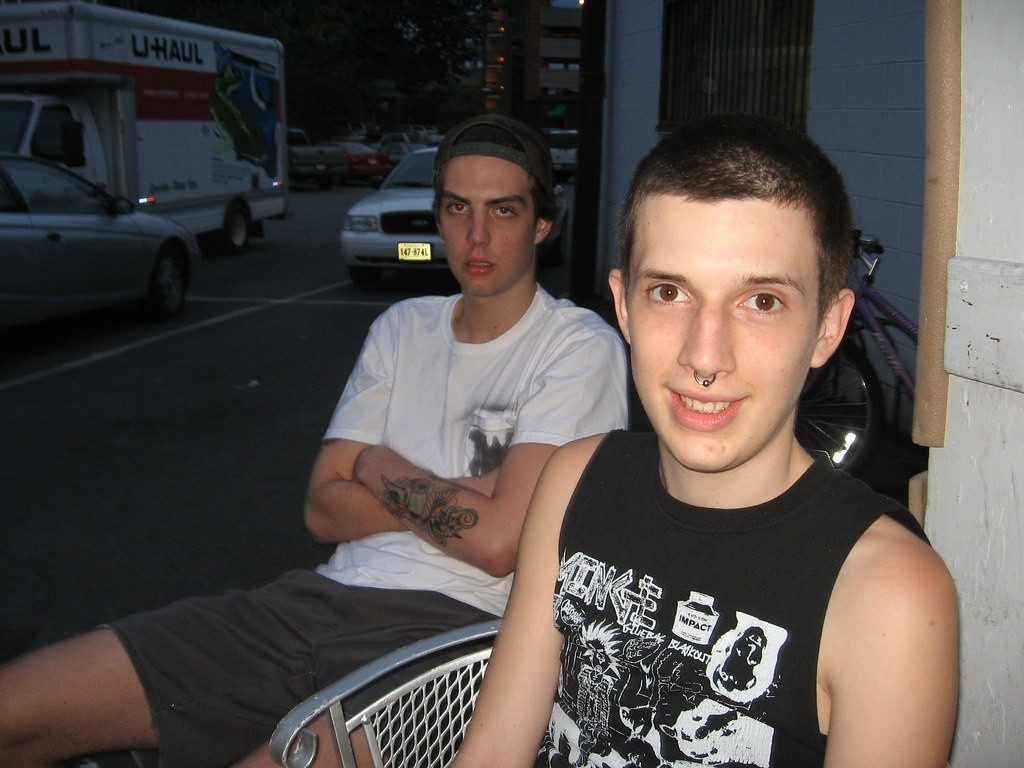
[0,0,294,254]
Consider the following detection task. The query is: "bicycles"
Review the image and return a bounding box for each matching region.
[795,231,919,478]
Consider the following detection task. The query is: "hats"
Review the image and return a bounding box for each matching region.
[433,115,557,205]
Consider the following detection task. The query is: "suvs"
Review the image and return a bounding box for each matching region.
[544,130,581,179]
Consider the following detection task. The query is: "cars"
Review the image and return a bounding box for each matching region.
[341,147,570,293]
[321,124,446,178]
[0,152,202,332]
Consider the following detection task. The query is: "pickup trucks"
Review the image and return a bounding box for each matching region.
[287,129,348,193]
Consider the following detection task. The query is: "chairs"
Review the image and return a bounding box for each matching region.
[269,618,507,767]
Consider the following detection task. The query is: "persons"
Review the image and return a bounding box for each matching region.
[0,105,629,768]
[453,110,959,768]
